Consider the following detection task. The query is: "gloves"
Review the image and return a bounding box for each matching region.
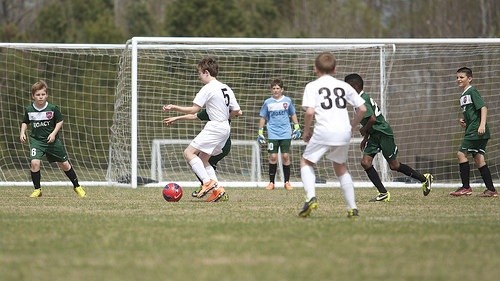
[258,128,266,146]
[290,123,301,139]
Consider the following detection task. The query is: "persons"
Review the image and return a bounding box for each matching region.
[450,67,499,197]
[163,57,242,201]
[20,79,87,198]
[344,73,433,202]
[258,79,302,190]
[299,52,367,218]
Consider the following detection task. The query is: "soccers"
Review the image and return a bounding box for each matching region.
[163,183,183,202]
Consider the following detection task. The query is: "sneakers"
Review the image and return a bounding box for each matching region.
[299,197,318,217]
[198,179,218,199]
[476,190,497,196]
[449,186,472,195]
[284,181,293,190]
[204,186,225,202]
[30,188,42,198]
[346,209,359,218]
[421,174,433,196]
[218,194,228,202]
[368,191,390,203]
[266,182,274,190]
[74,186,88,198]
[191,184,204,197]
